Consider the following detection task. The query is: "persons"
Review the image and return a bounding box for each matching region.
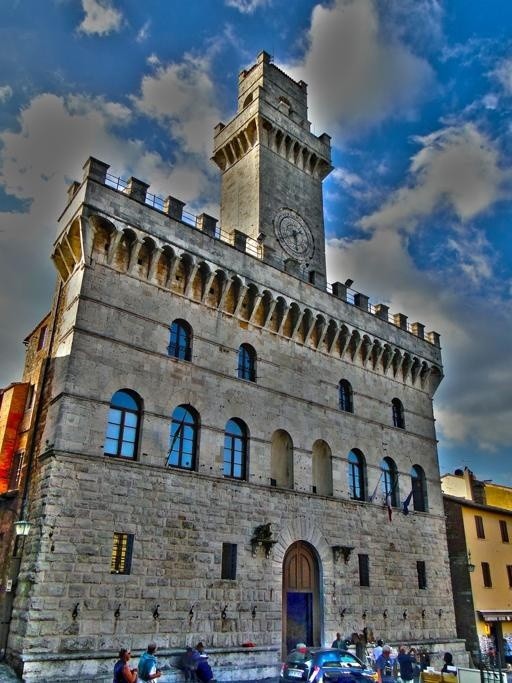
[136,640,162,682]
[184,643,217,682]
[487,646,495,667]
[331,631,348,651]
[181,641,204,682]
[439,650,457,677]
[504,638,511,665]
[371,636,428,683]
[113,645,139,683]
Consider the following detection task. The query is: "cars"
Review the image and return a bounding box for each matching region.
[276,645,381,683]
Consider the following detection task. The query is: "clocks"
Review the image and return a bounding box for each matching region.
[271,202,318,263]
